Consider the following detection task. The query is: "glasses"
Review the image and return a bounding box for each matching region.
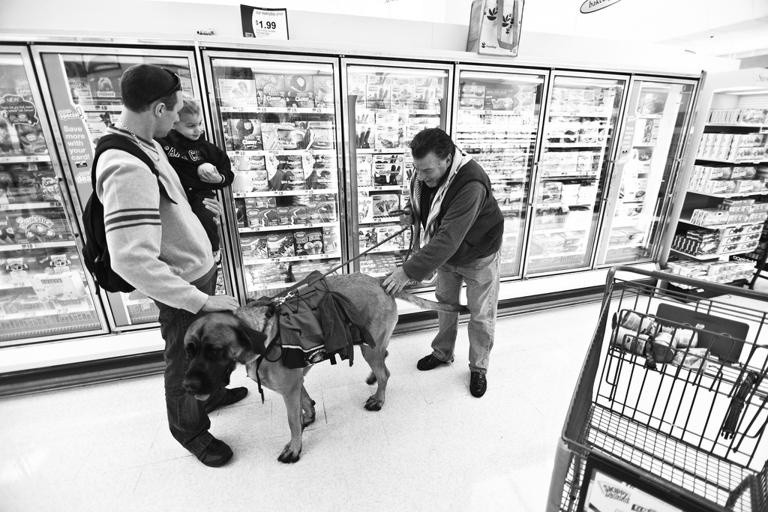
[145,66,183,104]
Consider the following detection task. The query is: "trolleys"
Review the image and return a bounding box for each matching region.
[544,264,766,512]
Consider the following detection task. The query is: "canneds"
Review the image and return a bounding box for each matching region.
[612,311,698,363]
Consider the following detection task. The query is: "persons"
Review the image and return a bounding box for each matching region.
[152,92,234,265]
[380,128,505,397]
[92,64,248,468]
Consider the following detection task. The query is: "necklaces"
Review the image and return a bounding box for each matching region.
[120,125,160,161]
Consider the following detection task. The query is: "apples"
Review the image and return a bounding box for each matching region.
[198,163,214,176]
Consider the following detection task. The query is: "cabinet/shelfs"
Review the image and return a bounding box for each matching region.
[0,36,768,400]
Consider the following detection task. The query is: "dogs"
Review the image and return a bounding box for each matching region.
[175,271,471,464]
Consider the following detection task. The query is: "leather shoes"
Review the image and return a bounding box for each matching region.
[195,435,234,469]
[417,353,455,371]
[469,372,487,398]
[196,386,249,414]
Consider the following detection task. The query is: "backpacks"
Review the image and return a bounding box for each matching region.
[80,188,138,296]
